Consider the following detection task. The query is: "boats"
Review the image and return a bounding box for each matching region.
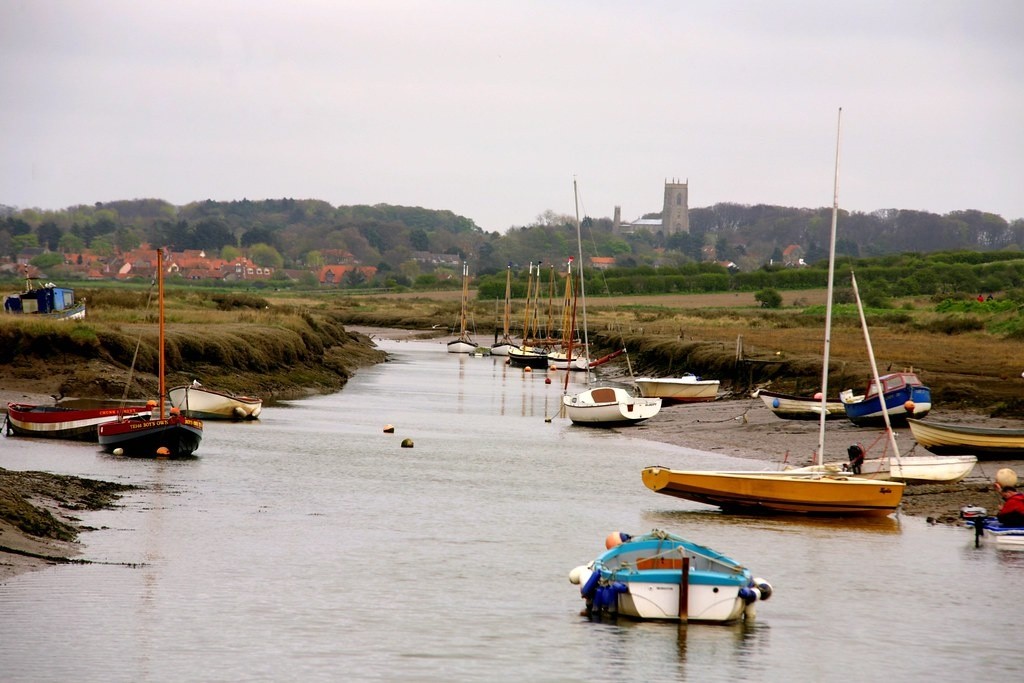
[642,465,906,518]
[562,387,662,425]
[751,389,845,420]
[168,379,263,420]
[0,404,152,439]
[635,372,720,402]
[906,418,1024,461]
[960,504,1024,551]
[839,366,931,428]
[569,527,773,626]
[3,264,85,321]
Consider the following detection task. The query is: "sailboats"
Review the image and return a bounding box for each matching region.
[491,256,592,369]
[98,248,203,460]
[799,108,978,484]
[447,262,478,353]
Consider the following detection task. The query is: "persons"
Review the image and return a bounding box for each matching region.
[977,294,993,302]
[993,483,1024,530]
